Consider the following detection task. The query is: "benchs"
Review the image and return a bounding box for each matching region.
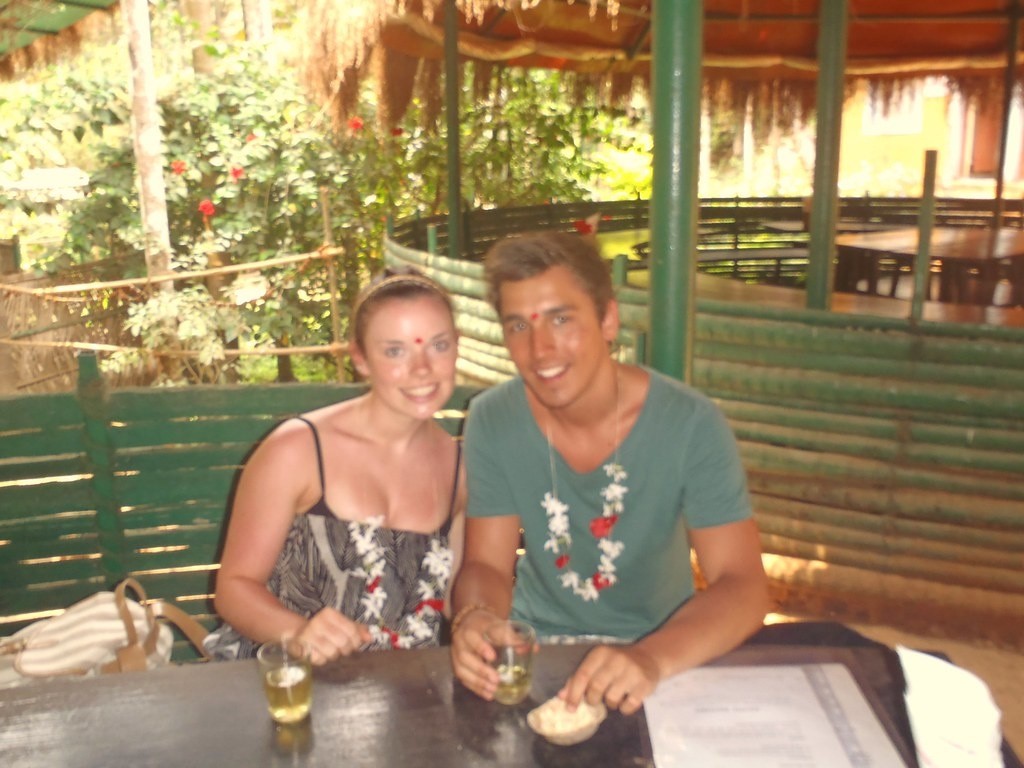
[697,233,1024,303]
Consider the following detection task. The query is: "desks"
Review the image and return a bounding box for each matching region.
[0,647,1022,768]
[764,218,913,232]
[834,221,1024,303]
[694,271,1024,330]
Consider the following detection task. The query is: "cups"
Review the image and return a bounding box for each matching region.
[256,636,314,725]
[482,621,537,704]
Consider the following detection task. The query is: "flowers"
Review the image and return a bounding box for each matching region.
[348,513,454,649]
[543,463,628,600]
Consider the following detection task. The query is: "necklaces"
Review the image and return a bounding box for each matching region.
[547,370,621,588]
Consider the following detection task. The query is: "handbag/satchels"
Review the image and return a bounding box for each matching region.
[0,578,214,688]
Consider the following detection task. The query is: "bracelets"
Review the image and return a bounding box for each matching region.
[451,602,495,635]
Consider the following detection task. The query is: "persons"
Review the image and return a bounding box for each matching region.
[202,269,468,669]
[451,232,770,717]
